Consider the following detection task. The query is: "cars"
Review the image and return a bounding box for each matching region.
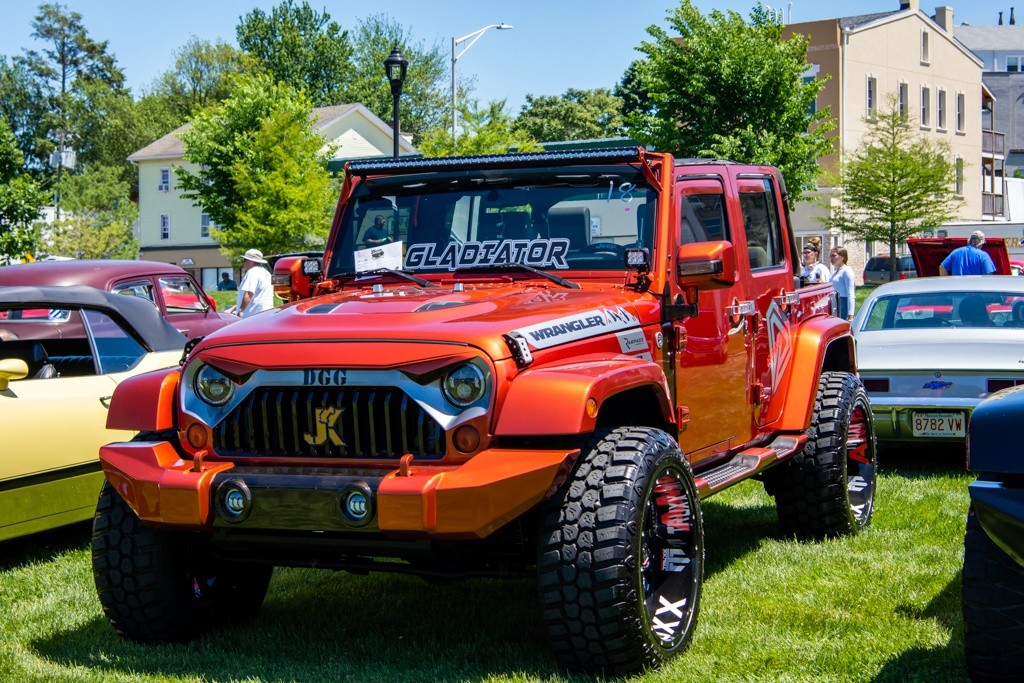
[894,235,1024,326]
[848,272,1024,464]
[0,285,198,546]
[862,251,919,288]
[0,258,242,373]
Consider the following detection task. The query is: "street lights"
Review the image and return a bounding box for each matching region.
[383,39,410,160]
[450,22,514,157]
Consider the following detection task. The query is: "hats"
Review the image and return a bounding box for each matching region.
[222,273,229,277]
[240,248,268,263]
[967,230,985,247]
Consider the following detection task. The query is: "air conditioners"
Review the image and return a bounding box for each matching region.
[158,184,168,192]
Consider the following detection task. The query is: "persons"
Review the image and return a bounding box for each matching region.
[829,246,855,320]
[958,295,995,327]
[224,249,273,318]
[217,272,237,290]
[939,231,997,276]
[362,215,392,248]
[803,237,830,284]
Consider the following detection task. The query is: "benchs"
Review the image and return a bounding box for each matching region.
[48,356,137,376]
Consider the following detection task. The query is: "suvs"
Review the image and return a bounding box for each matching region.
[88,137,882,682]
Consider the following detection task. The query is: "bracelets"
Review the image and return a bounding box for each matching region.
[238,308,244,312]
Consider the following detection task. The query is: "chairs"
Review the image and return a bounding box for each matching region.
[481,213,532,240]
[547,206,590,250]
[638,204,647,241]
[1004,301,1024,327]
[895,319,942,327]
[0,343,48,376]
